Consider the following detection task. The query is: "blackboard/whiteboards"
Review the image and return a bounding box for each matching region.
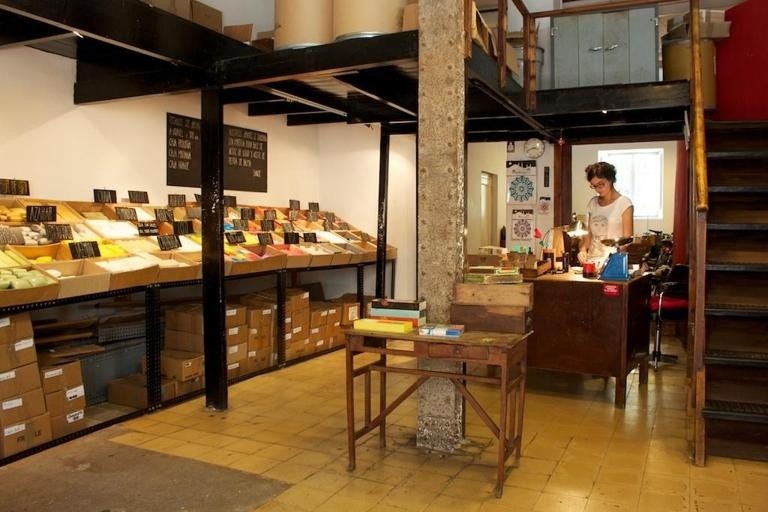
[166,112,267,192]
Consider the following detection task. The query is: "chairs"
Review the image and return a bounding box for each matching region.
[648,263,690,372]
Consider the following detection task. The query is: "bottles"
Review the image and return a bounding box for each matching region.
[562,253,569,272]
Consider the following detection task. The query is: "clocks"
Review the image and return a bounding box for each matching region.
[523,137,545,158]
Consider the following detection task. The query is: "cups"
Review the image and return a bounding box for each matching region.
[583,262,597,279]
[542,247,557,269]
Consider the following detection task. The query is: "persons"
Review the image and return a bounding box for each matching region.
[577,161,634,267]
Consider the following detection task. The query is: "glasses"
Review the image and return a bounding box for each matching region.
[590,180,606,188]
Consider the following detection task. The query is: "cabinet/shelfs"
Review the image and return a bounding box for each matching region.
[550,2,658,88]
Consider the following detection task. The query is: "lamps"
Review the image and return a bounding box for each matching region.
[536,219,590,263]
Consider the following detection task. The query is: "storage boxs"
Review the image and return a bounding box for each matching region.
[19,198,83,223]
[106,281,383,409]
[37,259,111,298]
[0,267,58,307]
[65,198,398,292]
[3,310,88,456]
[0,197,29,222]
[0,222,56,250]
[6,242,72,265]
[142,0,274,54]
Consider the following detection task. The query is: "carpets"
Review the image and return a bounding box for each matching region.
[0,424,295,512]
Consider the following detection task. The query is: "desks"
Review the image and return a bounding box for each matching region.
[340,321,535,499]
[524,266,653,407]
